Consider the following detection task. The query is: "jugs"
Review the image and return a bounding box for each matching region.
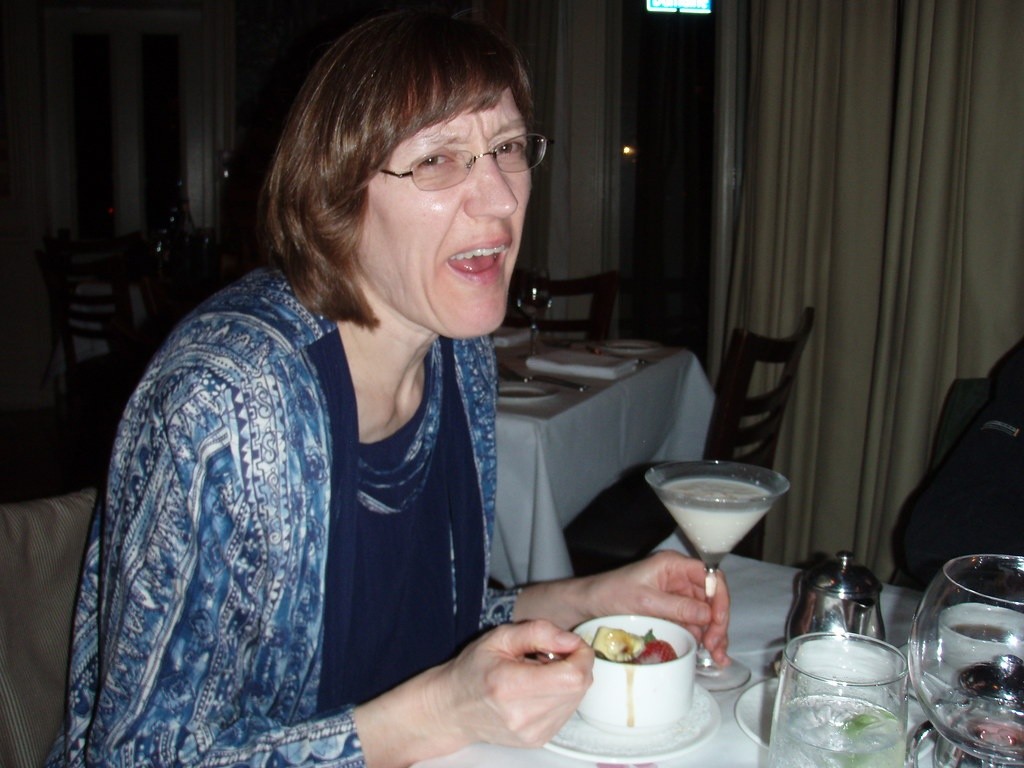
[905,719,1024,768]
[788,549,886,651]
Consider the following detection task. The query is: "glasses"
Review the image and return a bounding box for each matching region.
[379,134,554,192]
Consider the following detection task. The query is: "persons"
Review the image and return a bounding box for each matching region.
[43,13,729,768]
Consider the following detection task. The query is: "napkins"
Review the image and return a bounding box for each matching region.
[525,349,636,380]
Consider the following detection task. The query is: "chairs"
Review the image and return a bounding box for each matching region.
[0,487,97,768]
[889,379,990,591]
[36,226,214,478]
[502,265,614,338]
[564,307,815,572]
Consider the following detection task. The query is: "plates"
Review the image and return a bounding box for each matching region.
[497,382,560,406]
[735,677,783,749]
[535,684,720,764]
[600,340,660,355]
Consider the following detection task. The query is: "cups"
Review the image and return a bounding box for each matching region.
[766,633,909,768]
[909,554,1024,768]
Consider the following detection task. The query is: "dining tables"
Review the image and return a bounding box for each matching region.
[490,327,716,589]
[406,553,936,768]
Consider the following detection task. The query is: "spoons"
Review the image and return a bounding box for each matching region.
[537,632,643,664]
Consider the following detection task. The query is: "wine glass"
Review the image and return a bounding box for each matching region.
[644,462,790,692]
[517,270,551,360]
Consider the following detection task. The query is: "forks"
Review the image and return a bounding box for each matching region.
[499,363,590,392]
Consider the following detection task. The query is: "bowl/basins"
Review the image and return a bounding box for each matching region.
[936,601,1024,676]
[569,614,698,730]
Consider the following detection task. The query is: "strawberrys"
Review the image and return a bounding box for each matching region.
[636,641,678,664]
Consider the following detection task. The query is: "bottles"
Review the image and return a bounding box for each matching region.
[155,198,216,285]
